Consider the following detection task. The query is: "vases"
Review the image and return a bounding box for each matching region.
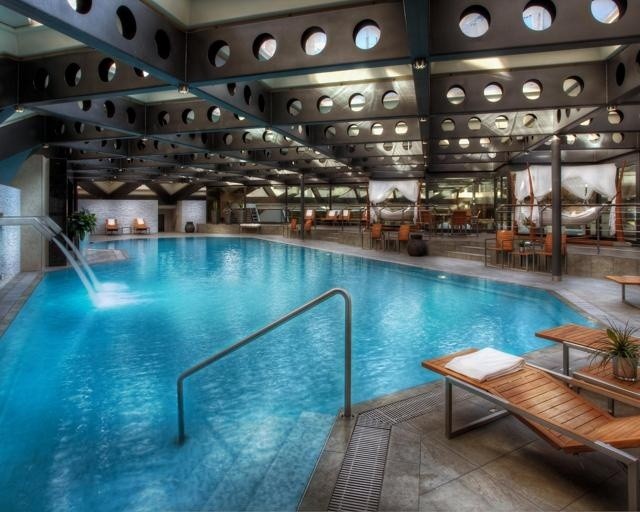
[184,221,195,232]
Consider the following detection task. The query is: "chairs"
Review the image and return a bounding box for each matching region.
[421,348,640,512]
[105,218,119,235]
[605,272,639,303]
[280,208,570,272]
[535,324,639,388]
[134,218,150,234]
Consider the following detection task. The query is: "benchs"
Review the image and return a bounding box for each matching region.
[239,223,261,233]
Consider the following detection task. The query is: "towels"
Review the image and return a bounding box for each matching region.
[445,348,524,383]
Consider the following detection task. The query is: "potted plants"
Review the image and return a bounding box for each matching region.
[591,322,640,383]
[65,203,100,261]
